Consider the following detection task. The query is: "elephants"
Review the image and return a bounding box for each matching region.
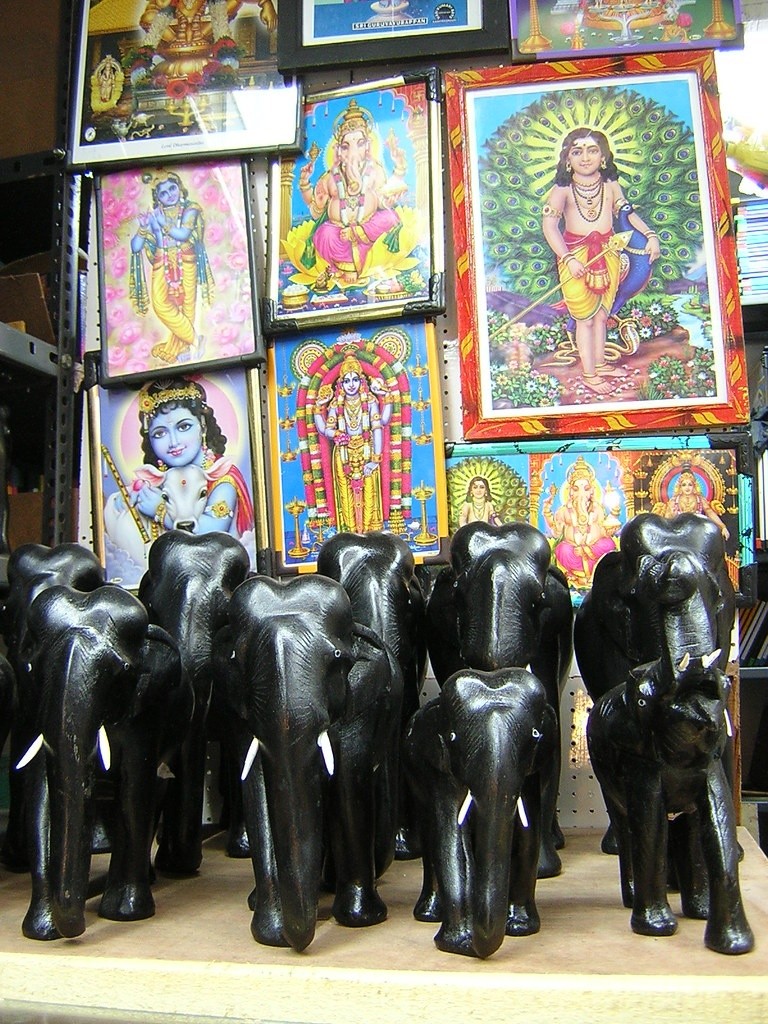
[575,512,755,958]
[214,532,431,951]
[403,520,575,958]
[2,542,202,942]
[137,531,248,874]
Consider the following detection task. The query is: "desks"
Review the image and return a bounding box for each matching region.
[0,826,768,1024]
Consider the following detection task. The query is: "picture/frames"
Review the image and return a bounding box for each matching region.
[258,65,447,336]
[274,0,511,76]
[263,315,452,578]
[445,48,753,443]
[67,0,308,175]
[509,0,746,66]
[90,156,268,390]
[446,430,762,610]
[82,348,276,594]
